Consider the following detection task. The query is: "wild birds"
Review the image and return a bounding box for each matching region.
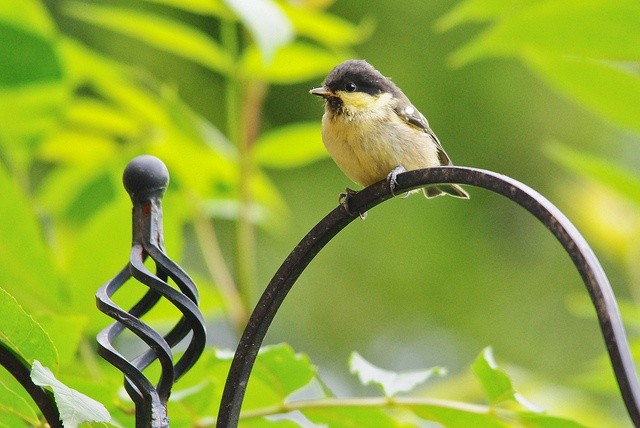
[308,60,470,199]
